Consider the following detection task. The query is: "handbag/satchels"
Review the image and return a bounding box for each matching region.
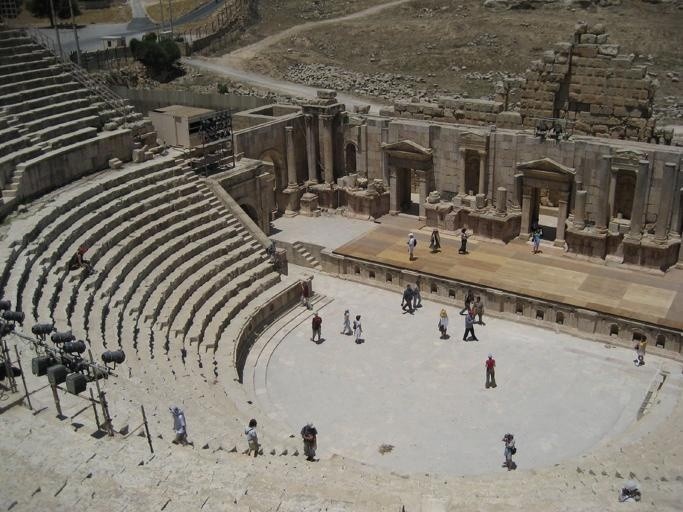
[512,448,516,454]
[439,325,443,331]
[414,239,416,246]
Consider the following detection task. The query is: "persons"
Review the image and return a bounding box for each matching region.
[406,233,415,261]
[459,289,474,315]
[633,337,647,366]
[400,283,414,314]
[168,405,187,446]
[300,280,309,309]
[429,229,440,253]
[531,228,542,254]
[616,478,642,504]
[472,297,484,324]
[244,418,258,457]
[352,315,362,343]
[68,245,97,274]
[299,421,317,462]
[457,228,471,254]
[484,353,496,388]
[309,311,322,342]
[437,309,448,337]
[340,308,352,335]
[462,309,478,341]
[412,279,422,307]
[502,432,515,470]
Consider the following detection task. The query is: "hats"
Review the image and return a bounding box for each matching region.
[408,233,414,236]
[308,422,314,429]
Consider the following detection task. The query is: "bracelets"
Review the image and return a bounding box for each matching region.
[629,493,631,497]
